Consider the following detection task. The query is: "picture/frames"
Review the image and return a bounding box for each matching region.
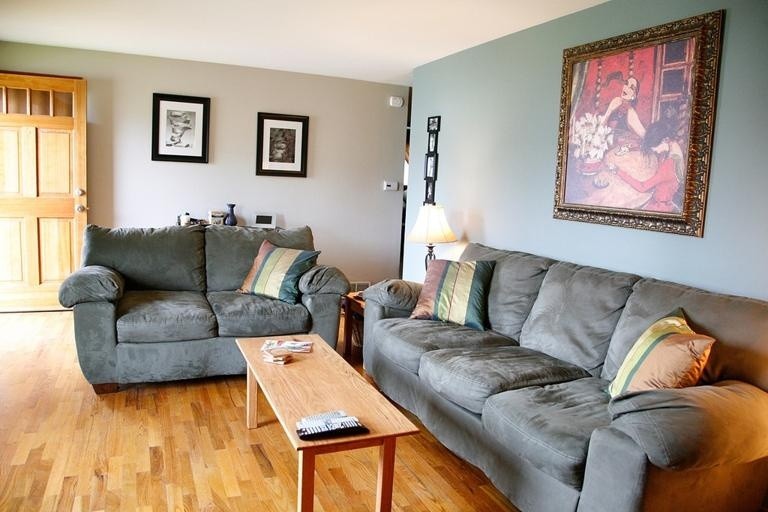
[151,93,211,164]
[256,112,309,178]
[552,8,727,239]
[422,116,440,206]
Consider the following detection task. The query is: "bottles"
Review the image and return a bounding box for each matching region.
[184,213,190,225]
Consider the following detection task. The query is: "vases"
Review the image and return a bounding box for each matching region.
[225,203,238,226]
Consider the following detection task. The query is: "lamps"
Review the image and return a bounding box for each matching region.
[406,205,458,271]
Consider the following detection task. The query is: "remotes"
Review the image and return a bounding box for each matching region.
[301,410,346,422]
[296,420,368,440]
[296,416,360,429]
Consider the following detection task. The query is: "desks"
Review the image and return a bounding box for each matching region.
[341,291,365,355]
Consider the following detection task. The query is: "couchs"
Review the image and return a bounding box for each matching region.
[356,243,768,512]
[58,223,351,395]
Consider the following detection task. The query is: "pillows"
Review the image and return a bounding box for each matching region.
[598,307,716,403]
[407,259,496,332]
[235,239,321,304]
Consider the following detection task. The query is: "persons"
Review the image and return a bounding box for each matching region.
[604,120,686,214]
[601,77,646,139]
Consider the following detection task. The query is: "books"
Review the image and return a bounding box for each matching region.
[261,338,313,353]
